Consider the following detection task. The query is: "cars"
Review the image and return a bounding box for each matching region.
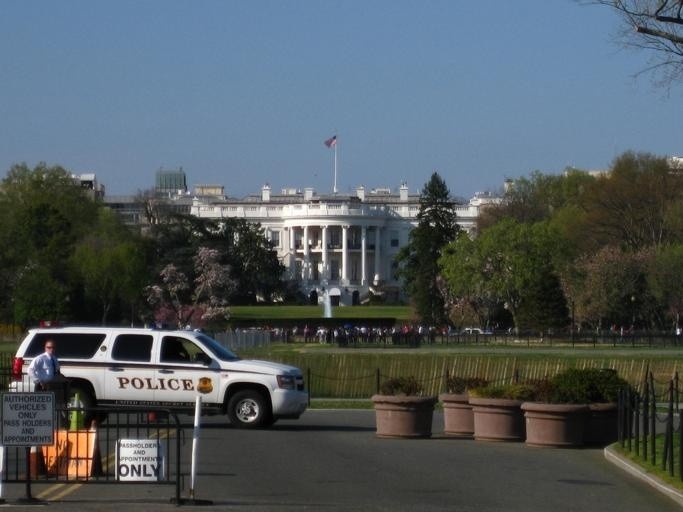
[8,320,309,429]
[463,325,494,335]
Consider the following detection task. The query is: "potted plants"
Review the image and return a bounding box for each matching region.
[371,367,641,449]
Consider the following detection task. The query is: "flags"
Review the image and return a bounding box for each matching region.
[323,135,336,148]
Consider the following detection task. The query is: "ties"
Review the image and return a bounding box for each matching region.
[51,357,56,375]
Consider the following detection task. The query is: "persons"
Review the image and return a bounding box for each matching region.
[25,339,65,395]
[227,325,453,347]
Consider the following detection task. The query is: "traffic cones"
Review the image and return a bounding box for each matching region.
[69,391,84,430]
[27,444,50,479]
